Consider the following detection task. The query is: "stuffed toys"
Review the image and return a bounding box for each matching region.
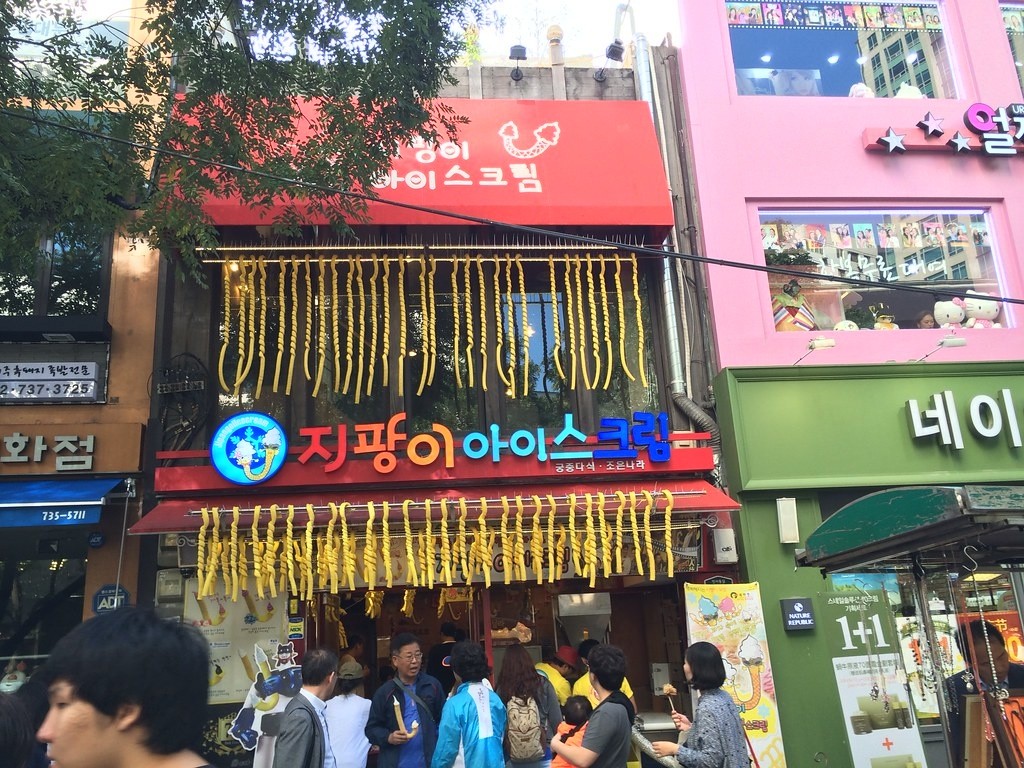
[964,290,1002,328]
[873,307,899,330]
[934,297,966,329]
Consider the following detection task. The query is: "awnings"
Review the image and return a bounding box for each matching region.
[0,479,126,526]
[127,479,743,538]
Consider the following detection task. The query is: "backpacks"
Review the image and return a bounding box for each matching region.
[505,692,547,763]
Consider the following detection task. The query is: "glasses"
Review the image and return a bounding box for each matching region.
[394,650,423,662]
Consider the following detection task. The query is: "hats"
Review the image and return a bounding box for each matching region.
[442,655,452,667]
[338,661,364,679]
[440,622,457,634]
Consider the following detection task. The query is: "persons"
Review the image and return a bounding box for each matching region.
[914,311,934,329]
[856,228,875,249]
[0,679,50,768]
[926,227,945,246]
[778,69,819,96]
[948,226,966,241]
[35,601,222,768]
[761,228,779,250]
[973,229,990,246]
[879,229,900,248]
[809,228,826,249]
[271,622,638,768]
[1003,15,1023,31]
[938,619,1024,768]
[836,227,851,247]
[652,642,751,768]
[902,223,921,247]
[729,4,941,29]
[784,229,802,249]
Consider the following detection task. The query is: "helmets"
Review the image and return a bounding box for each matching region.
[556,646,580,672]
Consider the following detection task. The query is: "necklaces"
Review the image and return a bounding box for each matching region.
[859,570,1008,743]
